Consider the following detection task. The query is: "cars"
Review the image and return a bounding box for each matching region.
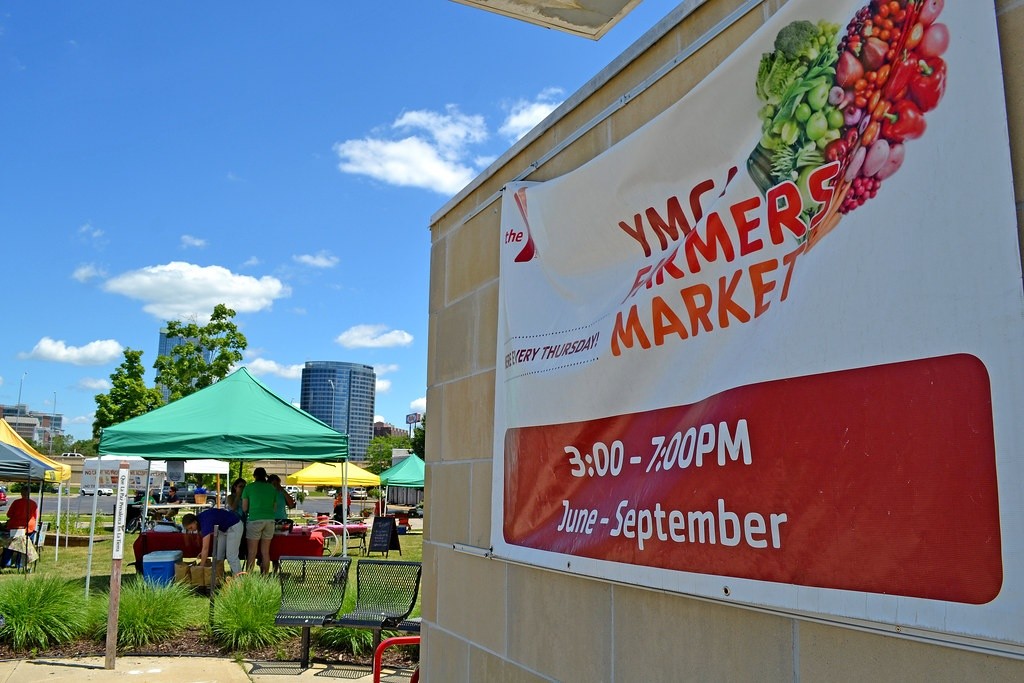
[62,453,83,457]
[280,485,309,497]
[350,487,367,500]
[327,490,337,497]
[0,485,9,506]
[78,487,113,496]
[132,478,217,508]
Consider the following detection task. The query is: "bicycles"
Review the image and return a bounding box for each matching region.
[127,506,156,534]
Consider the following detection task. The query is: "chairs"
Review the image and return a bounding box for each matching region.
[342,532,366,556]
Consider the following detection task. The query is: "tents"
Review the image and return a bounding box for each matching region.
[83,366,350,604]
[77,454,230,533]
[0,440,48,579]
[0,417,72,563]
[376,453,426,518]
[285,460,381,525]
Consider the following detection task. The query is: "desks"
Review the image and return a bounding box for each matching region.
[346,516,366,523]
[134,532,323,583]
[146,504,213,521]
[0,530,36,573]
[292,525,367,556]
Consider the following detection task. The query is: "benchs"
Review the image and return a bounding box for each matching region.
[273,556,421,674]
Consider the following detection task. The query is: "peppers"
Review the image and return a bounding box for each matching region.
[881,49,946,144]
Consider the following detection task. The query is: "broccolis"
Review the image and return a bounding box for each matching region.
[775,20,818,59]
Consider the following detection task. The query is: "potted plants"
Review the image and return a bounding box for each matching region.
[368,490,386,515]
[361,508,372,517]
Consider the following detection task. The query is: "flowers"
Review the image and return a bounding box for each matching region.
[195,488,205,494]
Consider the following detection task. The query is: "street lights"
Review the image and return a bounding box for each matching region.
[15,371,28,432]
[328,379,334,428]
[49,391,56,458]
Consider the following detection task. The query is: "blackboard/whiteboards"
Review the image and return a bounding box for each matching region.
[369,517,395,552]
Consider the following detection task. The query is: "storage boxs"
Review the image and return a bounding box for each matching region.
[143,551,182,585]
[205,561,223,584]
[174,559,194,587]
[191,564,204,587]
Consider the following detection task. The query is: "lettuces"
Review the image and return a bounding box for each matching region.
[755,51,807,103]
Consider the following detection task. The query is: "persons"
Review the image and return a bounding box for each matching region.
[332,498,351,525]
[374,495,381,518]
[156,487,180,522]
[193,484,206,495]
[228,478,247,519]
[0,486,39,568]
[141,488,157,521]
[346,492,351,508]
[380,497,388,515]
[241,466,278,576]
[255,472,294,574]
[182,508,244,580]
[333,490,341,509]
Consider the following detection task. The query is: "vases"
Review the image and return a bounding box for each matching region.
[195,494,206,504]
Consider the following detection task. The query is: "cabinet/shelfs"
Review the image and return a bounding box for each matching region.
[113,503,143,530]
